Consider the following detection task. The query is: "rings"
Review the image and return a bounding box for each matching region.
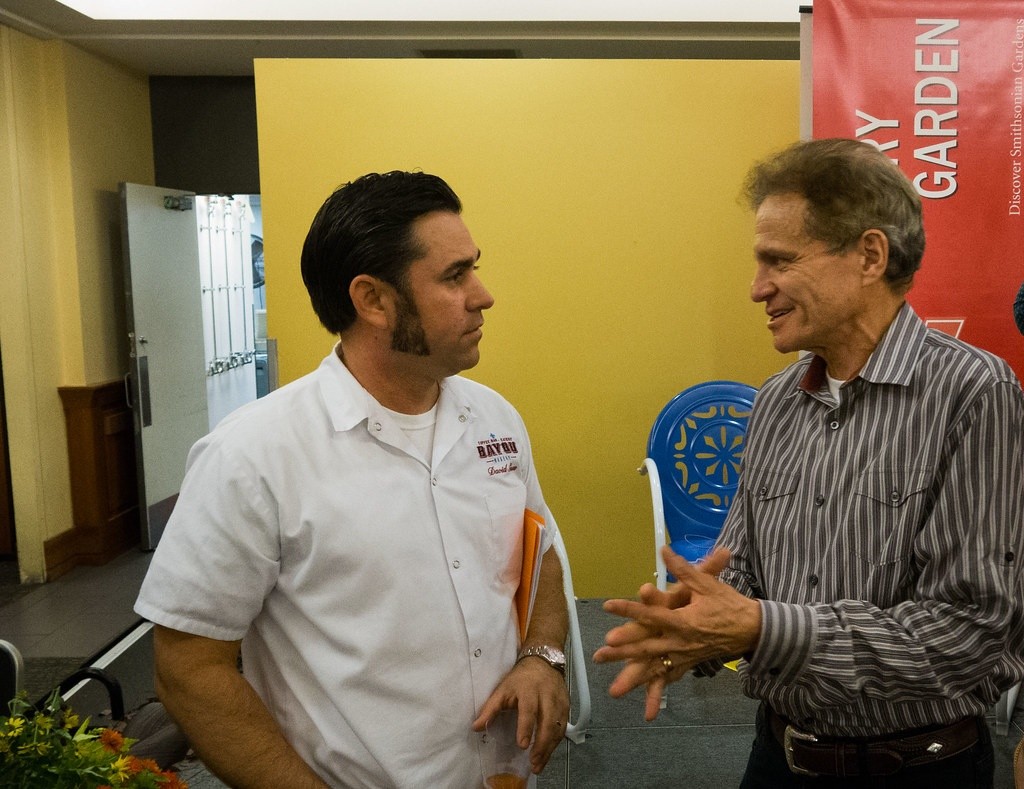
[661,656,675,672]
[557,721,563,727]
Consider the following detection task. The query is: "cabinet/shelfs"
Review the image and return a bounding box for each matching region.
[196,194,257,433]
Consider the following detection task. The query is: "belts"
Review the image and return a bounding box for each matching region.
[759,710,986,777]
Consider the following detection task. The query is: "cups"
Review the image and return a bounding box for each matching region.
[475,709,534,789]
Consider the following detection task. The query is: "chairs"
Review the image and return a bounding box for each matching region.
[637,382,759,710]
[0,639,191,771]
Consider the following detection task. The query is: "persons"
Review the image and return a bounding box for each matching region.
[134,170,569,789]
[594,139,1024,789]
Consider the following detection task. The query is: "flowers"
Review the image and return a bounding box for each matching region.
[0,686,190,789]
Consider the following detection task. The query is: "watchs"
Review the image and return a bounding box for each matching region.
[516,644,567,682]
